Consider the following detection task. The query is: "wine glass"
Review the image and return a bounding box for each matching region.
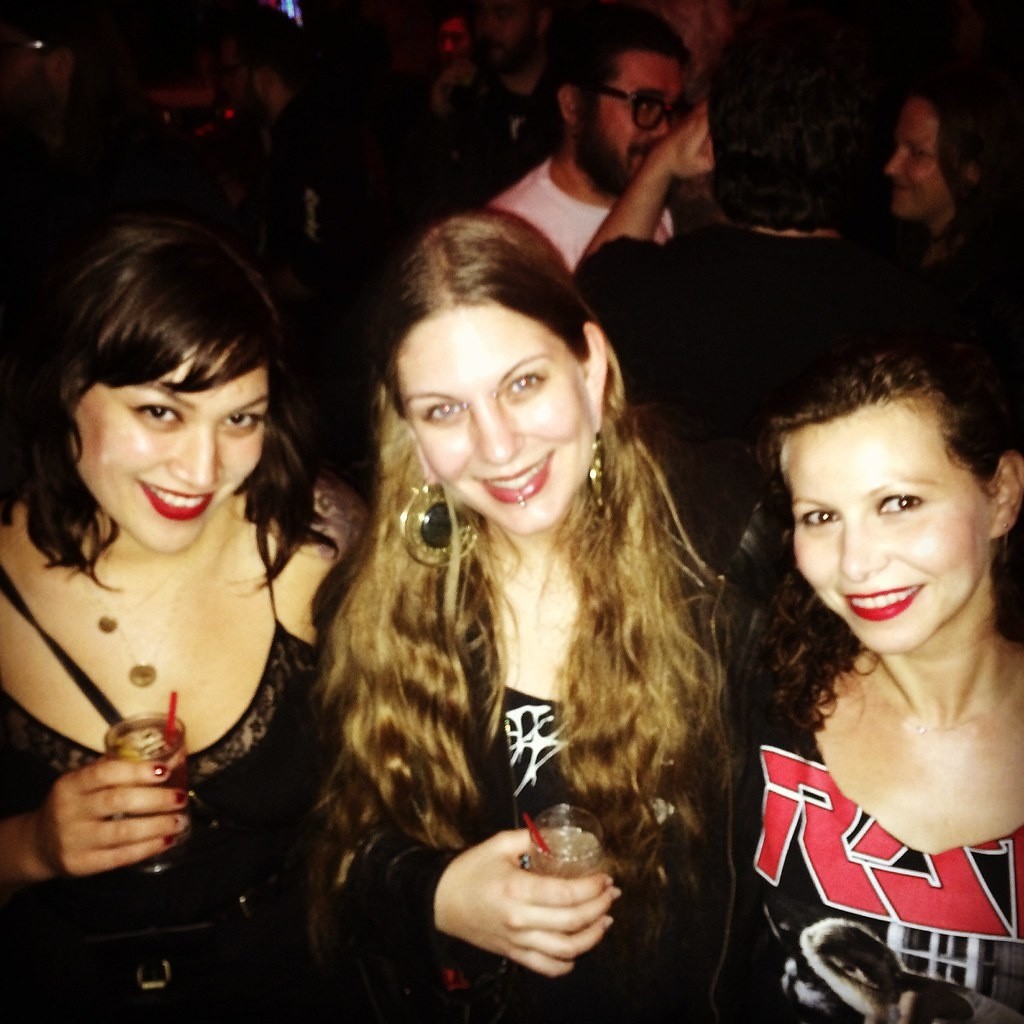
[103,711,188,872]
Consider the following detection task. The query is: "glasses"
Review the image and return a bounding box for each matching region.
[596,83,687,130]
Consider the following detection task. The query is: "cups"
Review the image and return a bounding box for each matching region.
[529,804,604,878]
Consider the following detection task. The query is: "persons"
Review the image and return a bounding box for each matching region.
[278,237,751,1023]
[678,319,1024,1024]
[0,208,376,1023]
[1,0,1023,518]
